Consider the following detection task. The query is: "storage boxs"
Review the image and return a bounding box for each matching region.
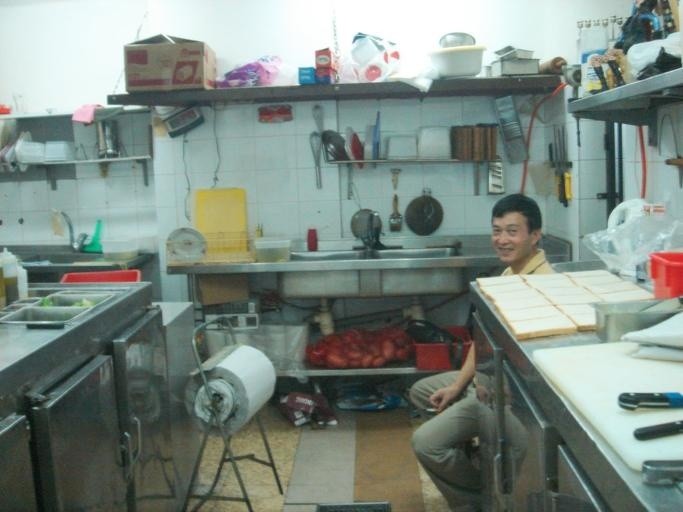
[60,269,141,282]
[125,34,217,95]
[409,326,471,371]
[649,251,683,298]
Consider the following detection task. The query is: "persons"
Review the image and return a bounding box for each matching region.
[406,193,554,511]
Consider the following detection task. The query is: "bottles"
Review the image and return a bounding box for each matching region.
[1,247,28,313]
[308,229,317,250]
[97,120,117,160]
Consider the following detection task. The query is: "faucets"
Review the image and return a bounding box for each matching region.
[369,211,379,244]
[61,212,74,244]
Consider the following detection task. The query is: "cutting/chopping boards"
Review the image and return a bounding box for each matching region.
[530,342,683,474]
[170,256,257,265]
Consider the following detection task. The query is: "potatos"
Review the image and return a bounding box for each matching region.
[310,330,407,368]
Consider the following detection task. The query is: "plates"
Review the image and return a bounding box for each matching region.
[165,226,208,262]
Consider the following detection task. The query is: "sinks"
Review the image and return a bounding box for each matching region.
[22,254,143,266]
[276,248,362,303]
[375,246,463,297]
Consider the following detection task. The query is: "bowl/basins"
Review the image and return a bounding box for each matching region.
[430,31,485,76]
[453,124,497,162]
